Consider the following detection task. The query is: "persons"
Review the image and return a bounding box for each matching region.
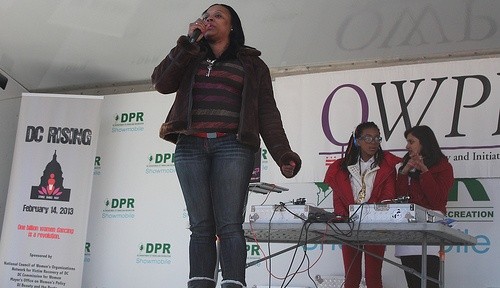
[393,126,453,288]
[151,3,302,288]
[324,122,402,288]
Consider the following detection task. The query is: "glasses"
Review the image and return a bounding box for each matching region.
[359,136,382,144]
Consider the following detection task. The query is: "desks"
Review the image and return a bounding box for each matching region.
[212,223,477,288]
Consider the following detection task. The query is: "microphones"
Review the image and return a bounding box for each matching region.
[190,28,201,43]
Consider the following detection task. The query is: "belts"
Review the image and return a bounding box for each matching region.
[191,133,228,138]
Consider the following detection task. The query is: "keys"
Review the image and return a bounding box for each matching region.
[205,58,216,77]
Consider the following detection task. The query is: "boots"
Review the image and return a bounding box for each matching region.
[220,280,245,288]
[187,277,216,288]
